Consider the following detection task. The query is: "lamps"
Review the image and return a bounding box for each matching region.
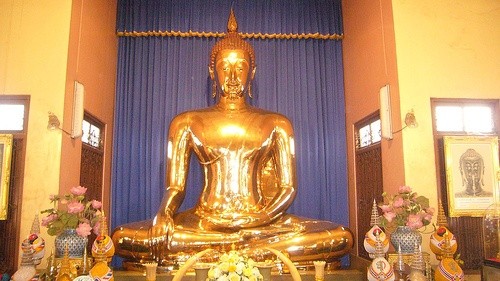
[378,82,394,142]
[70,80,87,140]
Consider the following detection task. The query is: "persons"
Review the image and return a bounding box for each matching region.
[453,148,494,198]
[112,9,356,273]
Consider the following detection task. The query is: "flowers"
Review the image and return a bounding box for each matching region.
[38,185,107,239]
[205,250,264,281]
[375,183,437,236]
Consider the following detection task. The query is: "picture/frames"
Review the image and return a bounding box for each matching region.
[440,134,500,219]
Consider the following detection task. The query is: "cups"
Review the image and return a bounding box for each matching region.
[145,263,158,281]
[257,266,272,281]
[313,261,326,281]
[193,268,210,281]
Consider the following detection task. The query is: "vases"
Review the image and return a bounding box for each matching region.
[55,226,89,257]
[389,224,424,253]
